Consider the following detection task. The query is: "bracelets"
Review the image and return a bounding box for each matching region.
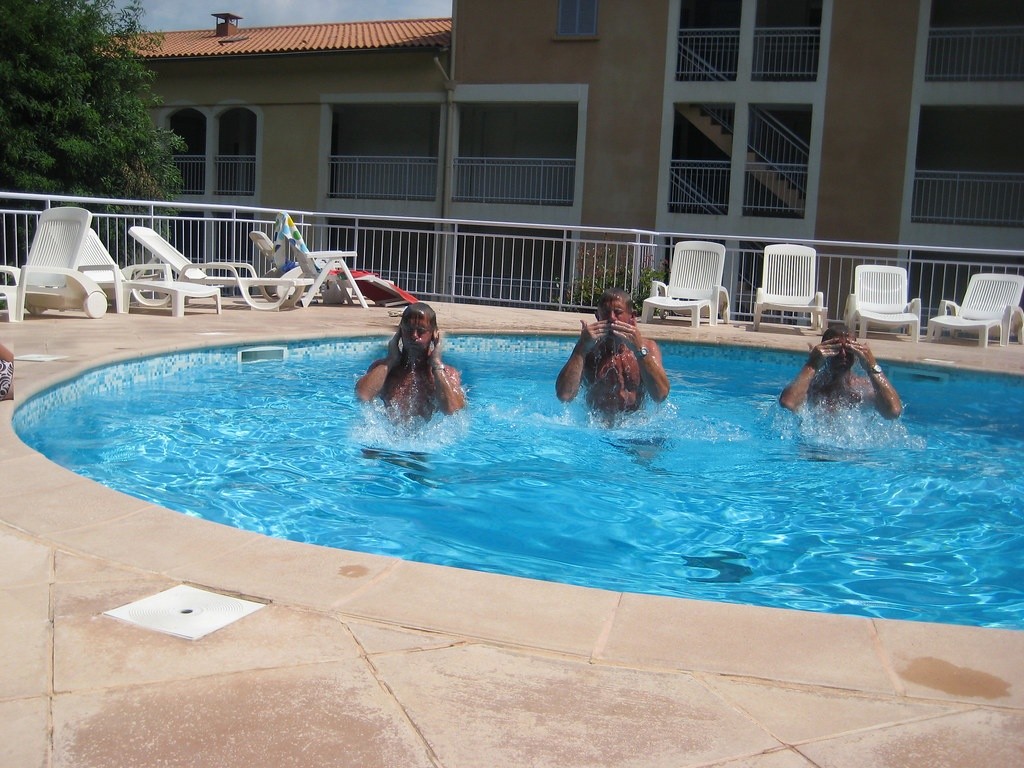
[431,363,446,371]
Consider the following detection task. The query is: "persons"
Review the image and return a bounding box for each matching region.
[780,313,903,421]
[354,302,465,424]
[555,287,670,418]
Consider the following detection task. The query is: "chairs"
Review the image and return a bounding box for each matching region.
[753,244,831,336]
[926,273,1024,349]
[0,206,420,323]
[641,240,732,329]
[843,264,921,343]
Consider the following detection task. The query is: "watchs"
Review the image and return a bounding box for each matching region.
[867,364,885,374]
[634,346,650,357]
